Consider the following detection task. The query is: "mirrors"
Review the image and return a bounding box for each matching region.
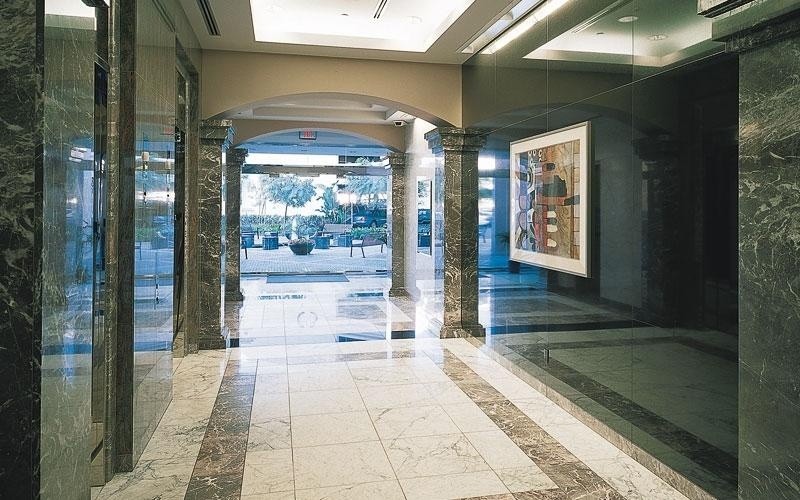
[415,175,433,257]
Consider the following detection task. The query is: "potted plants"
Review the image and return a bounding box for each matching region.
[287,238,316,256]
[495,228,521,274]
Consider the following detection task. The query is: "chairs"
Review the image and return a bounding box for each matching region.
[350,237,366,258]
[135,240,142,260]
[240,240,248,259]
[478,224,488,244]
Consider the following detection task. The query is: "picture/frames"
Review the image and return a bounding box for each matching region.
[508,120,595,280]
